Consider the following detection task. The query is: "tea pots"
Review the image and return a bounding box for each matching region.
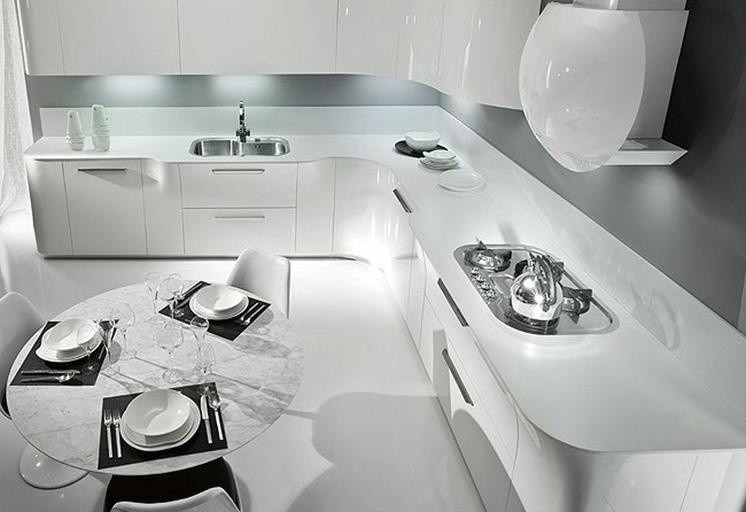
[510,250,564,321]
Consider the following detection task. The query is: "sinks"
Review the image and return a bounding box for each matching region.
[189,136,289,156]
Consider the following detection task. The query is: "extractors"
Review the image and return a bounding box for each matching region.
[520,0,690,172]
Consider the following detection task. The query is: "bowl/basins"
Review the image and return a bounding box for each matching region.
[40,318,100,350]
[196,284,243,310]
[404,131,440,151]
[126,389,190,437]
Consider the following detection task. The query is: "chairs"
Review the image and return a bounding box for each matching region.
[97,456,244,511]
[230,246,291,320]
[0,290,91,489]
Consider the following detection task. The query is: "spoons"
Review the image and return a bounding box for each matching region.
[207,392,224,441]
[173,283,204,300]
[20,372,74,383]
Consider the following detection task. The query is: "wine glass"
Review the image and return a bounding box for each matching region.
[155,319,184,382]
[144,271,185,320]
[77,324,97,370]
[109,304,137,360]
[190,318,212,375]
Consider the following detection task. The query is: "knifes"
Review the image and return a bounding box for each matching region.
[21,369,81,375]
[198,395,213,444]
[172,284,207,311]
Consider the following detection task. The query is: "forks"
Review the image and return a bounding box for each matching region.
[235,302,265,326]
[102,407,122,459]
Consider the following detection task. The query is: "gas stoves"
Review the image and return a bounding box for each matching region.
[454,238,623,339]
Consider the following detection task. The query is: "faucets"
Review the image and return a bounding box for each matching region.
[233,100,251,142]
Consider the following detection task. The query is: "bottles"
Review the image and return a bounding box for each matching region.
[65,109,85,150]
[89,104,110,151]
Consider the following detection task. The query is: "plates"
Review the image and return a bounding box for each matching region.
[188,288,249,320]
[34,322,103,362]
[439,168,484,193]
[422,149,455,164]
[417,158,460,170]
[394,139,448,159]
[117,393,202,452]
